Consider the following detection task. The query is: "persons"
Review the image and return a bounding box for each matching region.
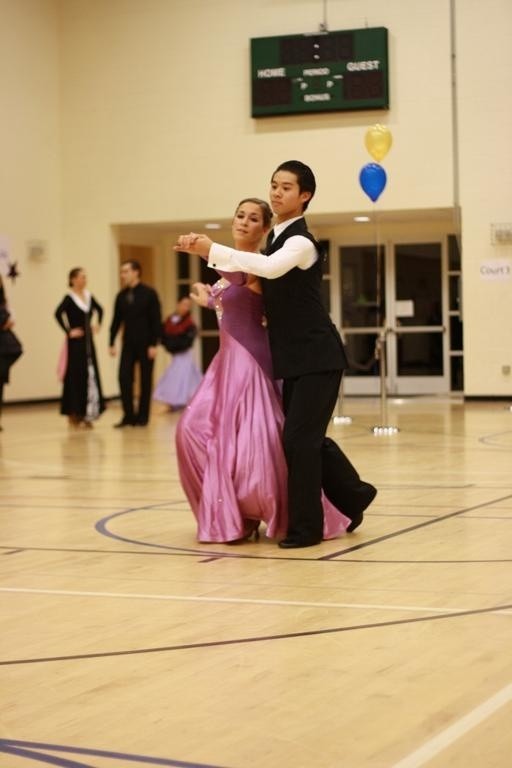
[150,295,204,412]
[52,268,107,432]
[1,271,24,432]
[108,259,163,430]
[175,197,352,545]
[174,160,379,549]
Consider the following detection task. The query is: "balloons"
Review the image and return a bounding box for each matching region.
[358,163,387,203]
[363,124,393,162]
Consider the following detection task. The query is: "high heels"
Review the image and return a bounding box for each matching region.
[229,520,261,544]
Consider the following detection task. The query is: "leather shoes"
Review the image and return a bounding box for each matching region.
[348,484,378,532]
[280,539,306,548]
